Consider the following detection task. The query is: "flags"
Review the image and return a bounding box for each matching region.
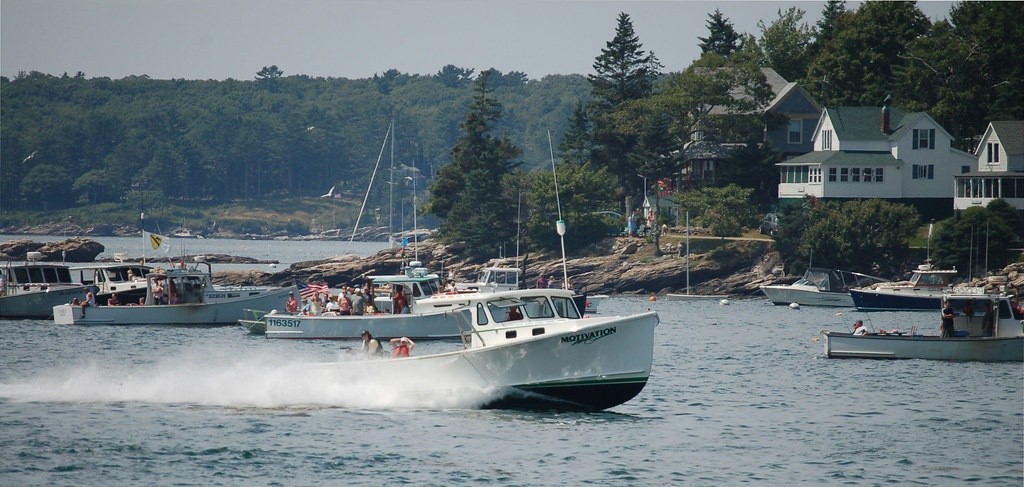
[295,278,328,300]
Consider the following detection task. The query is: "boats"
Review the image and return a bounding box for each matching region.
[759,248,891,307]
[237,117,610,343]
[0,209,303,327]
[820,294,1024,364]
[299,128,661,413]
[847,218,1005,312]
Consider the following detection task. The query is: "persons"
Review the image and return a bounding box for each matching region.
[79,286,94,318]
[562,278,574,290]
[390,337,415,358]
[299,284,373,317]
[108,292,121,306]
[166,280,177,305]
[359,330,383,356]
[850,320,868,336]
[1012,301,1024,320]
[536,274,555,288]
[982,306,993,337]
[286,291,297,312]
[941,300,954,337]
[151,277,164,305]
[394,288,410,314]
[628,207,654,238]
[444,266,458,294]
[505,306,521,320]
[0,270,7,296]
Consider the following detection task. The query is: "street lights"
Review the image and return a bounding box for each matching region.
[637,173,647,220]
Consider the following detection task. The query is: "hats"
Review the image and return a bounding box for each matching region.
[360,330,369,335]
[401,338,407,342]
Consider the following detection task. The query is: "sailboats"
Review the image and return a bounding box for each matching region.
[666,211,729,302]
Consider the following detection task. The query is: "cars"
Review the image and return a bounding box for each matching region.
[758,212,782,238]
[594,210,624,219]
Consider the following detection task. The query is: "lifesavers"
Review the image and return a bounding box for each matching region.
[447,292,457,294]
[964,304,972,313]
